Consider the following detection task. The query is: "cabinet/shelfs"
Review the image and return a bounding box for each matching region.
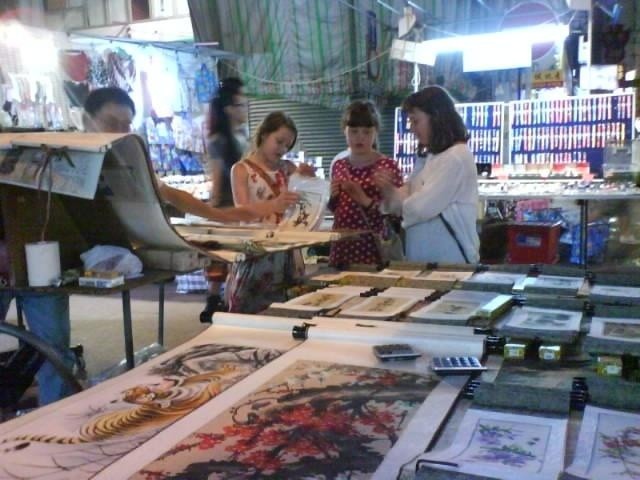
[393,178,640,268]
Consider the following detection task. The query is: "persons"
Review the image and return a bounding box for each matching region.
[222,110,319,318]
[195,88,251,322]
[374,83,482,266]
[326,99,407,270]
[0,86,276,409]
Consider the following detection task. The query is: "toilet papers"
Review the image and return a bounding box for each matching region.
[25,240,62,286]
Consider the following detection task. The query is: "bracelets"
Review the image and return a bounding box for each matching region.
[367,198,375,210]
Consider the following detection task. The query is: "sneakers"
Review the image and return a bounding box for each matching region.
[200,295,228,322]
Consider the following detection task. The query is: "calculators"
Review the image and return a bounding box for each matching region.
[373,344,422,361]
[430,356,487,374]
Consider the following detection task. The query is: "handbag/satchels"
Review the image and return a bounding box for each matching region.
[375,231,404,265]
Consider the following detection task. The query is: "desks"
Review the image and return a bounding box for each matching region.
[0,229,370,369]
